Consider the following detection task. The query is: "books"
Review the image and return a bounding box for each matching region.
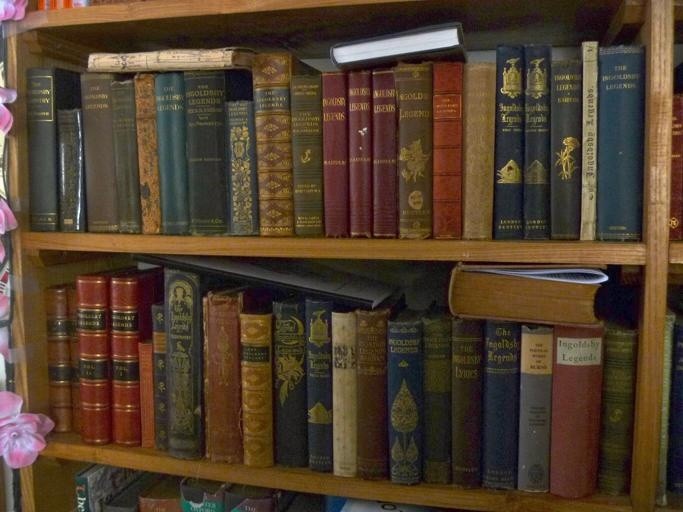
[76,463,441,512]
[42,252,636,497]
[661,317,683,507]
[24,22,645,242]
[668,92,683,242]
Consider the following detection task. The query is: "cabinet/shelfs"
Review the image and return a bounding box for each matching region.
[4,1,682,512]
